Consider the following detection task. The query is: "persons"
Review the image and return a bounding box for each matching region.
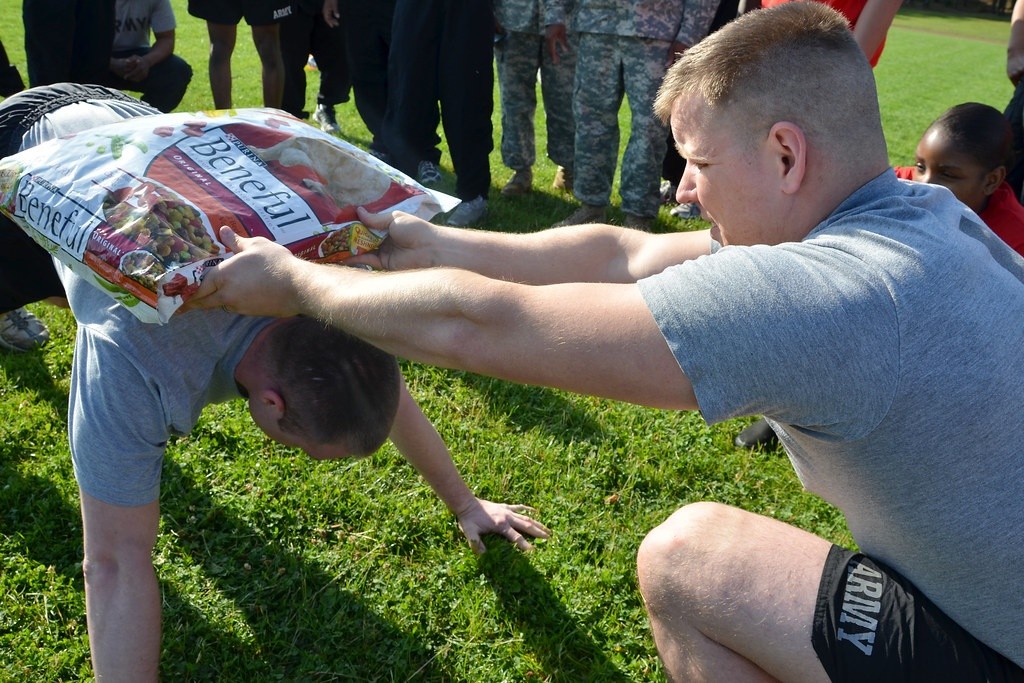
[0,82,551,683]
[1001,0,1024,206]
[0,0,900,229]
[735,102,1024,451]
[177,0,1024,683]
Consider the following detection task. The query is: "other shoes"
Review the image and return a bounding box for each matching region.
[735,419,776,448]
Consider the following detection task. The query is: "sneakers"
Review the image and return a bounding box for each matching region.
[624,215,653,235]
[368,149,385,161]
[552,164,573,194]
[551,202,607,229]
[312,103,339,134]
[501,168,532,198]
[447,196,487,227]
[417,160,440,187]
[671,202,699,220]
[658,180,678,204]
[0,307,49,353]
